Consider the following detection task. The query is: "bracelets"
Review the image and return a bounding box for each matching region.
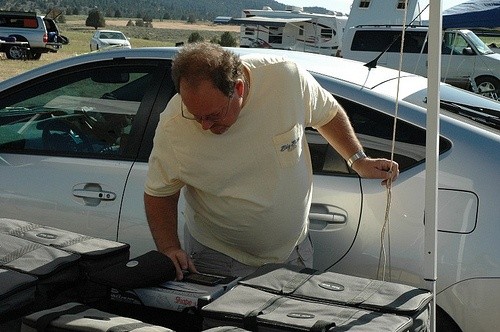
[347,150,366,167]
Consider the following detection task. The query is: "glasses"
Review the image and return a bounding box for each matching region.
[180,91,232,120]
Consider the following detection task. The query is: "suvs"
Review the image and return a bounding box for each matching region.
[0,8,69,61]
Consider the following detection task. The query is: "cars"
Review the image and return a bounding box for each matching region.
[90,29,132,50]
[0,46,500,332]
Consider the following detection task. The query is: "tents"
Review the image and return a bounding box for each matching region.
[414,0,500,83]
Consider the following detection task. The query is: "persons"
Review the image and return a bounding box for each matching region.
[143,41,400,279]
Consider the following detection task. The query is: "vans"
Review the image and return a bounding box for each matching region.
[341,23,500,103]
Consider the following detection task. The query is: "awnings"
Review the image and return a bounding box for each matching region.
[228,15,313,33]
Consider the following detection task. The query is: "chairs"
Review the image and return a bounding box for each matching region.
[100,34,121,39]
